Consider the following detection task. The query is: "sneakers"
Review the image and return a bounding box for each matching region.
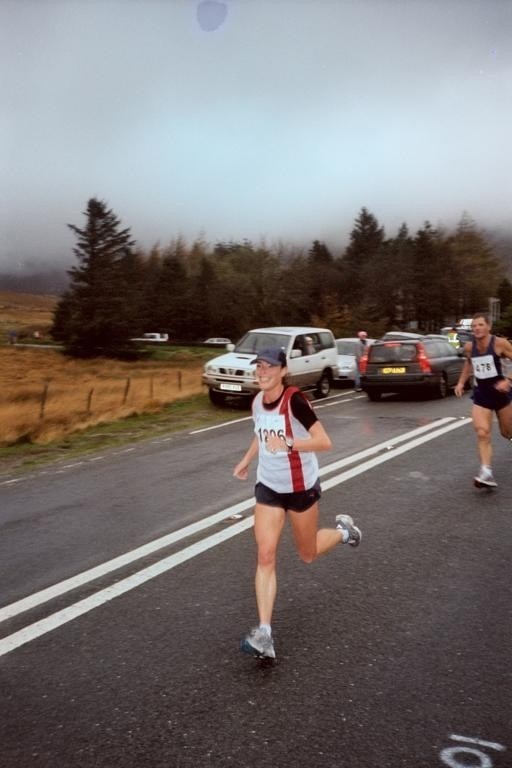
[473,473,498,489]
[335,514,362,548]
[243,627,276,665]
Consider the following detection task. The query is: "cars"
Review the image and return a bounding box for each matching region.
[128,333,169,342]
[202,325,476,405]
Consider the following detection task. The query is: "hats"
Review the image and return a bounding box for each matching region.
[357,331,368,336]
[250,347,287,365]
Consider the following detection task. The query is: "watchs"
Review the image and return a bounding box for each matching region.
[286,439,294,454]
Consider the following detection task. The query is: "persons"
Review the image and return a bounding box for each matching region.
[447,328,461,350]
[305,335,313,354]
[232,347,363,662]
[353,330,368,392]
[454,312,512,488]
